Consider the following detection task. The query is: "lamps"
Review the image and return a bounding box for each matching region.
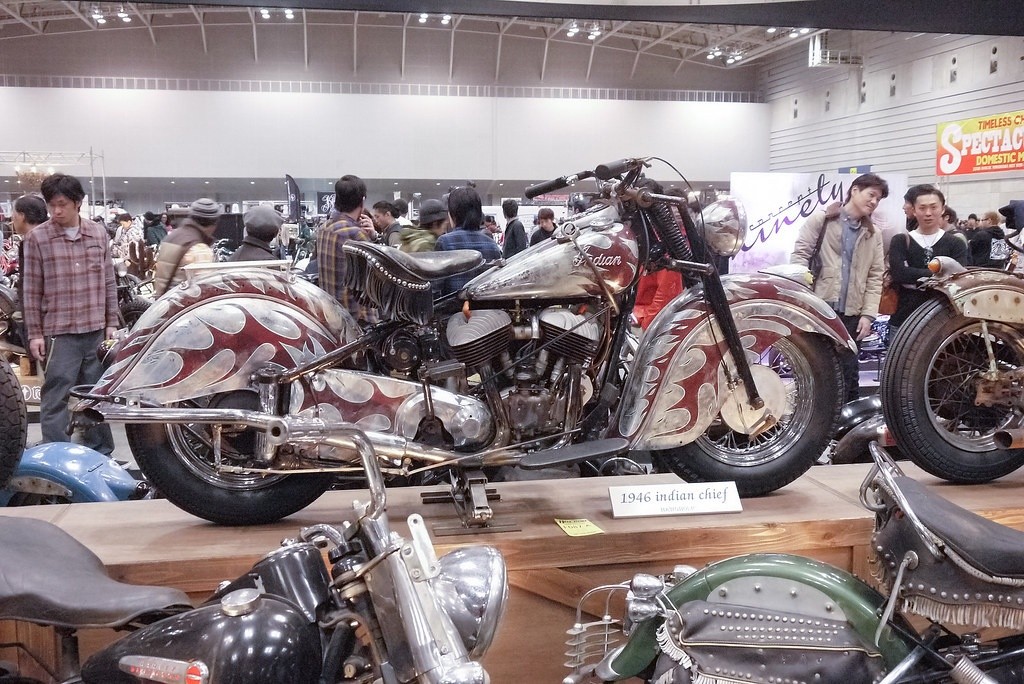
[260,7,295,19]
[765,25,810,38]
[419,12,452,25]
[707,44,748,64]
[90,6,133,24]
[567,19,602,40]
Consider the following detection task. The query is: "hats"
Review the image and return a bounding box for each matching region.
[420,199,448,224]
[243,206,283,236]
[189,198,221,217]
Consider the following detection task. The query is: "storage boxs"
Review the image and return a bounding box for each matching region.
[0,462,1024,684]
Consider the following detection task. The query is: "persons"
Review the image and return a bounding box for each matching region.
[502,200,526,261]
[903,184,935,231]
[788,174,885,403]
[435,186,501,291]
[92,211,172,259]
[529,208,557,247]
[359,198,500,254]
[573,200,586,214]
[316,174,383,324]
[888,187,968,343]
[11,194,50,358]
[227,205,284,262]
[968,211,1011,270]
[963,214,977,238]
[938,207,967,246]
[23,173,128,468]
[154,198,221,302]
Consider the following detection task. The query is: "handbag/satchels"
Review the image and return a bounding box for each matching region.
[808,254,823,290]
[879,258,899,315]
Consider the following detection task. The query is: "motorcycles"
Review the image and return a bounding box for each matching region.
[878,201,1023,486]
[560,436,1024,684]
[0,417,508,684]
[70,154,863,526]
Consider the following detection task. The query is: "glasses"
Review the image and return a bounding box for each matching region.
[981,218,989,223]
